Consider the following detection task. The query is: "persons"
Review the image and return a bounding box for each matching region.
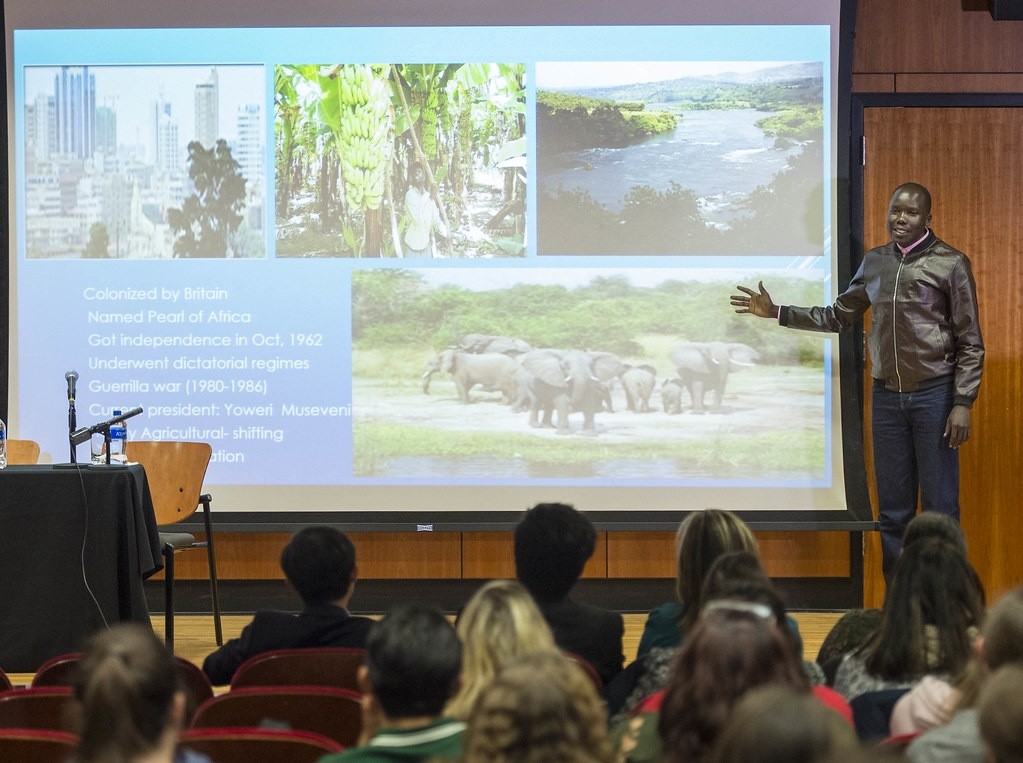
[403,164,448,258]
[309,581,618,763]
[817,510,1023,763]
[615,509,866,763]
[455,502,626,687]
[731,182,985,582]
[60,625,213,763]
[199,527,378,687]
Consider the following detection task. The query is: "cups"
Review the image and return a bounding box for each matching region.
[90,432,104,464]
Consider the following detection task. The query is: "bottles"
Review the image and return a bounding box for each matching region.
[0,418,7,469]
[110,410,128,465]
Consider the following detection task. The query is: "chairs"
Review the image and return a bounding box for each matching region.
[121,440,222,653]
[1,646,369,763]
[6,440,39,465]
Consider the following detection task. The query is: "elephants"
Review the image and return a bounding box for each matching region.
[667,338,761,413]
[660,378,686,415]
[617,363,656,413]
[422,333,628,436]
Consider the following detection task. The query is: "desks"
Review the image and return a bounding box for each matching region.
[1,462,164,671]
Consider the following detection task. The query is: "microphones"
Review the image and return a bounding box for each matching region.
[70,407,143,446]
[65,370,79,409]
